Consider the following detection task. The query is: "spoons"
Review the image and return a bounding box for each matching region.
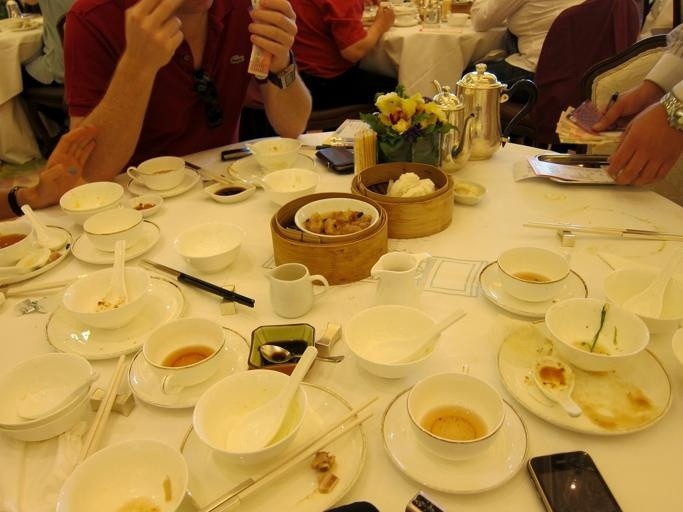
[621,246,682,319]
[533,358,583,416]
[382,309,467,364]
[223,347,320,449]
[17,369,99,418]
[260,345,345,366]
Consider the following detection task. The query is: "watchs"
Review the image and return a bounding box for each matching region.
[266,50,298,91]
[659,90,683,132]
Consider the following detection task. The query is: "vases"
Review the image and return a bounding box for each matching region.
[376,130,445,170]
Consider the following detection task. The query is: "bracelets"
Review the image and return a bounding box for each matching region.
[8,185,28,217]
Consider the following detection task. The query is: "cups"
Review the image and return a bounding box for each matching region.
[250,139,306,169]
[496,247,570,301]
[126,156,186,191]
[363,1,472,30]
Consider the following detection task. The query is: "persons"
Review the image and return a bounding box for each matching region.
[0,124,100,221]
[21,0,75,124]
[468,0,585,103]
[61,0,316,184]
[288,0,400,110]
[593,22,683,188]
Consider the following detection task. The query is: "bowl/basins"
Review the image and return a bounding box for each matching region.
[245,323,317,370]
[260,169,319,208]
[142,319,228,397]
[343,305,443,378]
[190,369,309,465]
[173,222,244,273]
[405,373,506,462]
[545,298,651,371]
[600,268,681,335]
[3,352,94,443]
[56,439,188,511]
[293,196,381,234]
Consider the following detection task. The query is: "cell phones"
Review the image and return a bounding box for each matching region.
[527,450,622,512]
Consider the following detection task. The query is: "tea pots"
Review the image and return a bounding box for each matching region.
[422,87,476,170]
[264,264,330,319]
[429,61,538,160]
[366,250,434,302]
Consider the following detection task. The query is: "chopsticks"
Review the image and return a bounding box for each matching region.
[198,396,378,511]
[522,220,682,242]
[143,256,255,309]
[185,160,232,185]
[74,356,128,468]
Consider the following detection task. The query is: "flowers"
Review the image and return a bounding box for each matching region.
[359,79,458,167]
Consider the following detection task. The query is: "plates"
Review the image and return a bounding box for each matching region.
[126,325,250,412]
[0,168,199,362]
[5,22,37,33]
[228,152,316,191]
[204,181,257,202]
[381,387,529,497]
[497,320,673,437]
[452,180,487,205]
[480,259,589,318]
[181,380,365,511]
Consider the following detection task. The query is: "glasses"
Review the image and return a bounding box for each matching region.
[192,71,222,129]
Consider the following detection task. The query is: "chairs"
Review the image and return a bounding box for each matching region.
[298,103,379,132]
[15,82,71,163]
[577,30,682,209]
